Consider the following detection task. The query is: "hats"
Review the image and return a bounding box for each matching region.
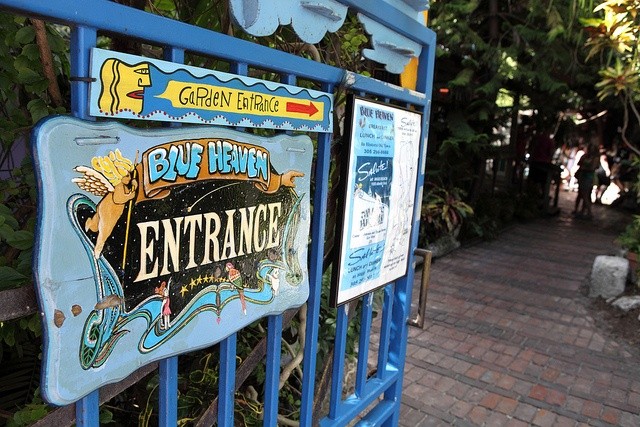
[590,144,600,155]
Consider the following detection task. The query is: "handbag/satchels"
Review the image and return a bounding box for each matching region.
[574,168,585,180]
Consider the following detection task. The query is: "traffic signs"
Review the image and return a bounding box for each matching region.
[91,48,334,134]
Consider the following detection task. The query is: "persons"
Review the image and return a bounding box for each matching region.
[595,155,610,203]
[552,141,601,220]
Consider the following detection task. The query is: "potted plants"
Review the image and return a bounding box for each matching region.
[615,214,640,273]
